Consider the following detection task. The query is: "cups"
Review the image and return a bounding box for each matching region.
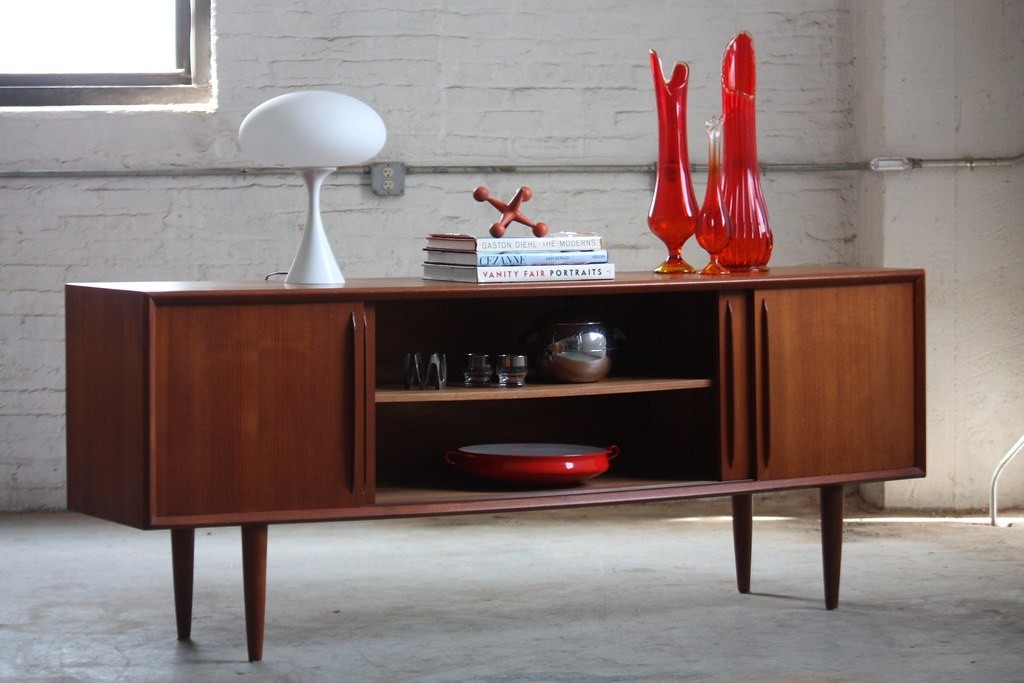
[496,354,528,388]
[463,353,493,387]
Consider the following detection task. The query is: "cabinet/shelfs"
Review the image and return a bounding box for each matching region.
[61,265,925,661]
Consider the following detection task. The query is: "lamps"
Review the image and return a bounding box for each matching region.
[238,91,386,284]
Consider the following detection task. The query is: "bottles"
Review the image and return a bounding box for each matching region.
[647,30,775,277]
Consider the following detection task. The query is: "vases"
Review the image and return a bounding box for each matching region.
[650,45,699,275]
[721,31,775,272]
[695,113,727,275]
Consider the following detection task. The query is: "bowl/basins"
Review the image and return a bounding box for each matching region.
[445,443,619,486]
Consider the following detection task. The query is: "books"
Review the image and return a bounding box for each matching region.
[421,232,615,283]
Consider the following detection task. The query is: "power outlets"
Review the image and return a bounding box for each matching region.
[370,162,408,195]
